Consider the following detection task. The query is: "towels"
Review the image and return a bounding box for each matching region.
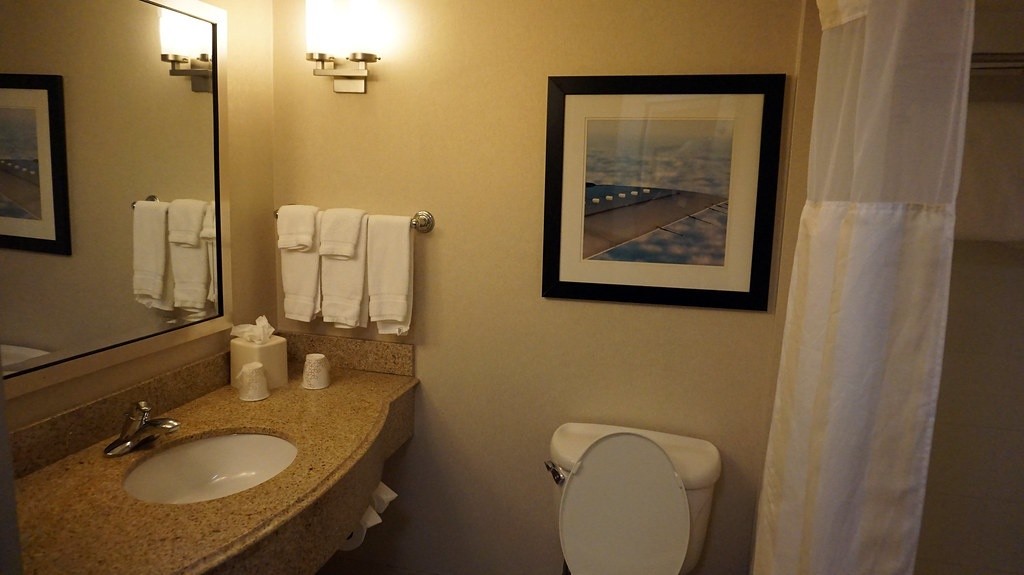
[131,200,175,312]
[319,209,369,330]
[275,205,323,323]
[200,200,219,305]
[366,215,417,336]
[168,198,210,321]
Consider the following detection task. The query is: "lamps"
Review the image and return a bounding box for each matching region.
[306,0,389,93]
[161,8,214,92]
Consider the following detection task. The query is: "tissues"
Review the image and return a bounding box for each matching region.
[228,314,289,389]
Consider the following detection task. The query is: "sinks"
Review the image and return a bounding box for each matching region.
[119,423,299,506]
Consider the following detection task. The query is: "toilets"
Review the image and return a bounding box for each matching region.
[544,422,722,575]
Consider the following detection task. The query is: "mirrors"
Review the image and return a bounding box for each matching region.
[0,1,218,378]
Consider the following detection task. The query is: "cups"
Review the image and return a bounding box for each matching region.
[238,363,273,402]
[303,354,332,391]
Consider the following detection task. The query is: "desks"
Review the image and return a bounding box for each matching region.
[0,360,421,575]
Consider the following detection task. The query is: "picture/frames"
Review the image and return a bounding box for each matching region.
[0,73,71,256]
[541,72,787,311]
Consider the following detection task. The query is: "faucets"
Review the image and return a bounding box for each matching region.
[103,401,182,459]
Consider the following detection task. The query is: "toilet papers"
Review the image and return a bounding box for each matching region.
[337,503,382,553]
